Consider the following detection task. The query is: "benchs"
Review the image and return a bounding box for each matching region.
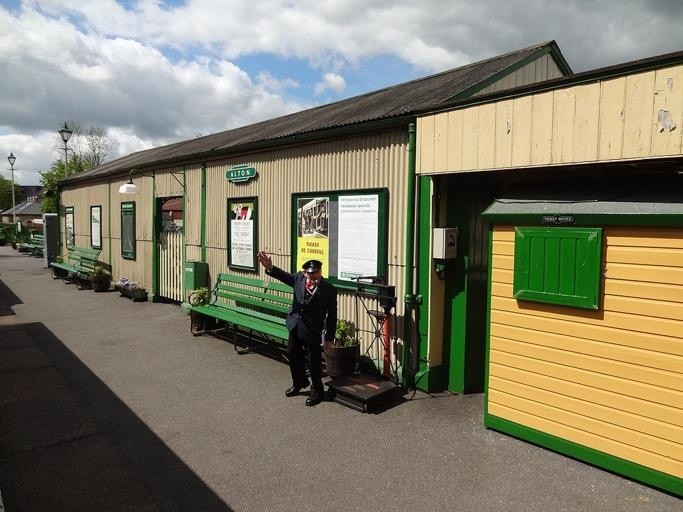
[22,234,44,258]
[189,273,294,354]
[50,246,100,289]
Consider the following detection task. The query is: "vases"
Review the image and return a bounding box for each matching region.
[119,287,148,302]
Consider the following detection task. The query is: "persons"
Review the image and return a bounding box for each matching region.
[254,250,337,407]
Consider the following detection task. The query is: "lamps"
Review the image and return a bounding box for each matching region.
[118,169,154,195]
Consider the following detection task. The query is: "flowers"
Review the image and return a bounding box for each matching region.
[115,278,141,290]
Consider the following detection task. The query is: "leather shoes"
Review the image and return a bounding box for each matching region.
[285,381,310,398]
[305,391,325,407]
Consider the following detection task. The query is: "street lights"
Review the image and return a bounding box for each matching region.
[58,121,73,177]
[6,151,16,224]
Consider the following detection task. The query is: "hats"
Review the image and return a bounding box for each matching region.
[302,259,323,273]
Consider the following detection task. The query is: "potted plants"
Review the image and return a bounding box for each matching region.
[325,319,359,379]
[91,266,113,292]
